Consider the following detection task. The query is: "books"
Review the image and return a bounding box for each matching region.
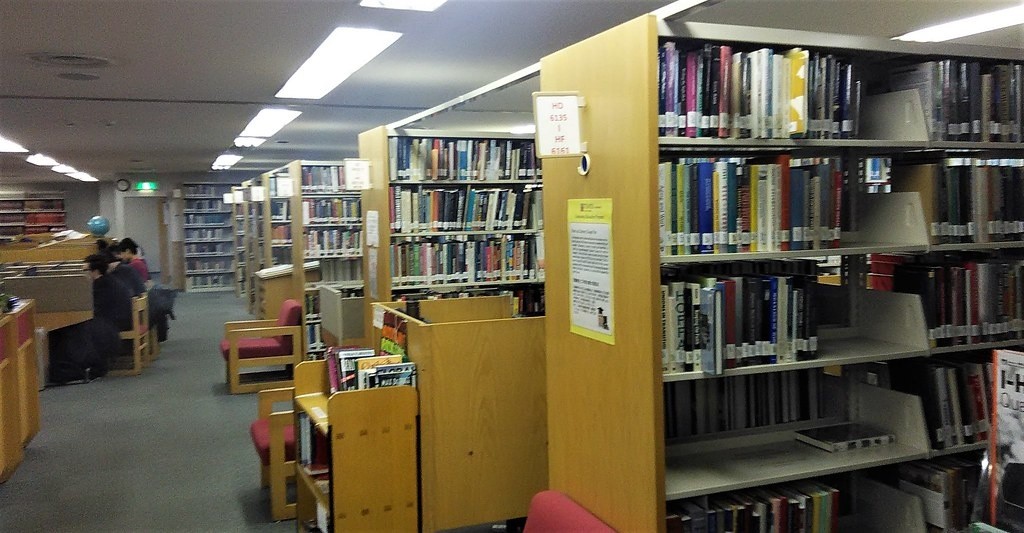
[186,184,253,292]
[301,348,416,533]
[0,200,66,235]
[256,165,363,348]
[388,139,543,319]
[658,39,1023,533]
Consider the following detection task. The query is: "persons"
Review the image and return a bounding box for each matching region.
[52,254,132,378]
[97,238,148,295]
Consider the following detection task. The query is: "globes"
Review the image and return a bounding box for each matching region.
[86,215,112,238]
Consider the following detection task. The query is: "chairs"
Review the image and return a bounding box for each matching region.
[522,490,618,533]
[249,386,297,522]
[221,298,303,396]
[106,292,160,378]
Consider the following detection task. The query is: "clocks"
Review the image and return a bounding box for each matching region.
[117,179,130,191]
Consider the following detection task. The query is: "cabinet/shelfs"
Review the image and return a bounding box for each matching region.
[370,294,549,533]
[292,360,419,533]
[319,284,364,350]
[357,124,546,356]
[537,13,1024,533]
[181,172,289,314]
[287,159,362,364]
[255,259,321,321]
[0,197,115,485]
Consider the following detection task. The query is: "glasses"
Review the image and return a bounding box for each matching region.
[81,267,91,272]
